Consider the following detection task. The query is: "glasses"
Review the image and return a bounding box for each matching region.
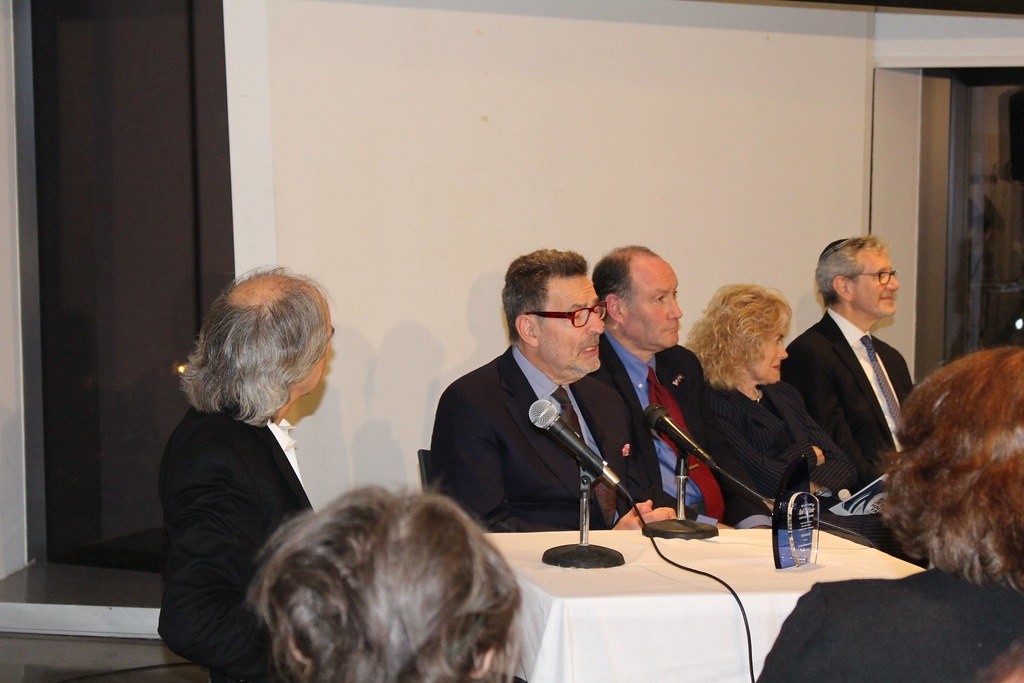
[847,268,899,286]
[526,301,607,328]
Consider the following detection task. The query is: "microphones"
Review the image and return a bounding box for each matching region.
[529,400,630,495]
[644,404,717,469]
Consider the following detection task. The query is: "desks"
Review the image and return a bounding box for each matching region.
[0,559,212,683]
[481,526,928,683]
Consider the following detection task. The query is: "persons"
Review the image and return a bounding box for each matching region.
[586,247,771,529]
[247,486,521,683]
[687,282,928,569]
[782,236,916,482]
[425,250,678,528]
[752,347,1024,683]
[156,270,336,683]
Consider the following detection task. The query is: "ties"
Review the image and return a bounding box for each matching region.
[645,365,725,522]
[861,336,904,429]
[551,387,616,527]
